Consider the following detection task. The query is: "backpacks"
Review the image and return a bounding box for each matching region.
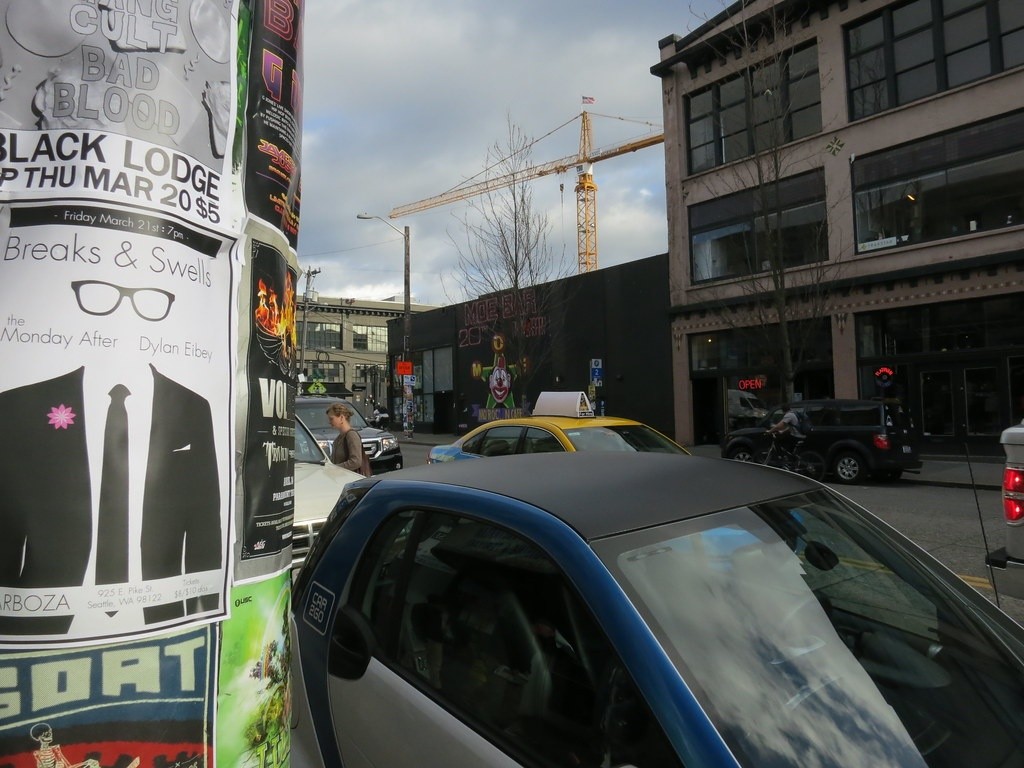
[785,411,815,435]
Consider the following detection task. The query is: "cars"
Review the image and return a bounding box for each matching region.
[983,420,1024,599]
[428,421,694,462]
[290,414,365,596]
[289,450,1023,767]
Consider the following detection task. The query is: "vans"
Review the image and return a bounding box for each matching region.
[727,389,768,428]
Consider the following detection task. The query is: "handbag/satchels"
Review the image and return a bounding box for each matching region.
[344,428,371,479]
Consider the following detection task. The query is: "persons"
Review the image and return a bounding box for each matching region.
[326,402,362,474]
[766,404,806,462]
[373,406,389,430]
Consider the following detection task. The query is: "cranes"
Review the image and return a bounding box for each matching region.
[390,109,664,272]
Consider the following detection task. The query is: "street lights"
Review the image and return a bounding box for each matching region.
[357,214,412,441]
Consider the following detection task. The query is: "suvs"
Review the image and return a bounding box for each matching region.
[719,399,920,486]
[294,391,404,476]
